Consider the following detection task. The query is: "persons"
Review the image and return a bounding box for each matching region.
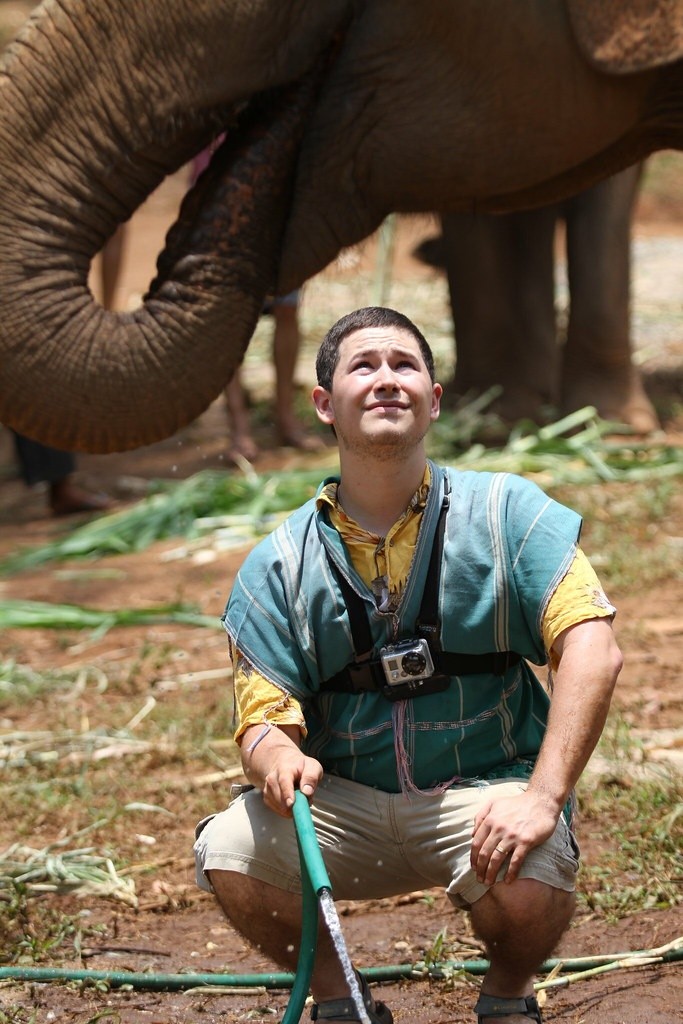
[192,307,624,1024]
[14,125,321,517]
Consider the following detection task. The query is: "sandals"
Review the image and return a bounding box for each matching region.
[310,969,393,1024]
[473,990,543,1024]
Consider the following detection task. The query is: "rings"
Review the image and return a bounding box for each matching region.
[496,845,511,857]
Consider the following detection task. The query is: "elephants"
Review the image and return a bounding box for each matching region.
[0,0,683,453]
